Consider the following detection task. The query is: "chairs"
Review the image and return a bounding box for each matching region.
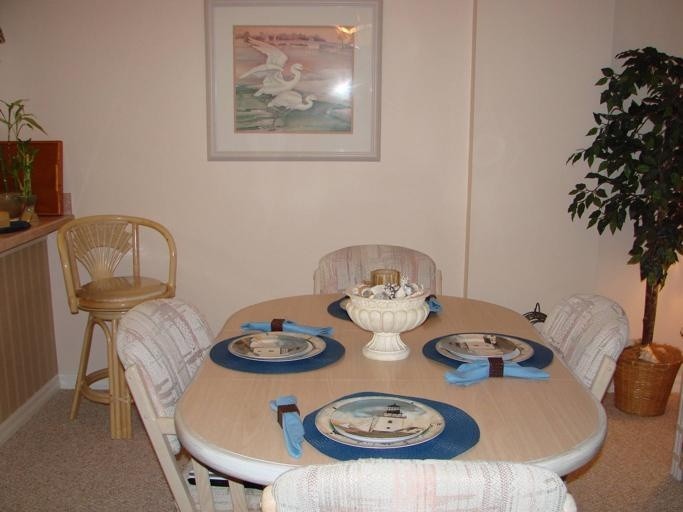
[314,245,442,296]
[113,296,261,512]
[57,214,177,441]
[539,292,628,402]
[262,458,575,512]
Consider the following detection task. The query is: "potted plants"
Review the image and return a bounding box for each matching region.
[0,109,48,228]
[567,48,683,416]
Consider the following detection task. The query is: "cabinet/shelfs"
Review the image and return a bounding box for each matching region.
[1,214,74,451]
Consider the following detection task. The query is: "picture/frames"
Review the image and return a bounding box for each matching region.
[203,0,383,162]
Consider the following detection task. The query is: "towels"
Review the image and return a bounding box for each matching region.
[424,294,442,313]
[442,357,551,387]
[269,394,308,460]
[240,318,333,337]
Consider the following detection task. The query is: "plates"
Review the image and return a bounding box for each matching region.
[313,396,445,449]
[330,398,430,443]
[227,332,327,362]
[435,332,534,366]
[443,334,517,358]
[231,334,313,358]
